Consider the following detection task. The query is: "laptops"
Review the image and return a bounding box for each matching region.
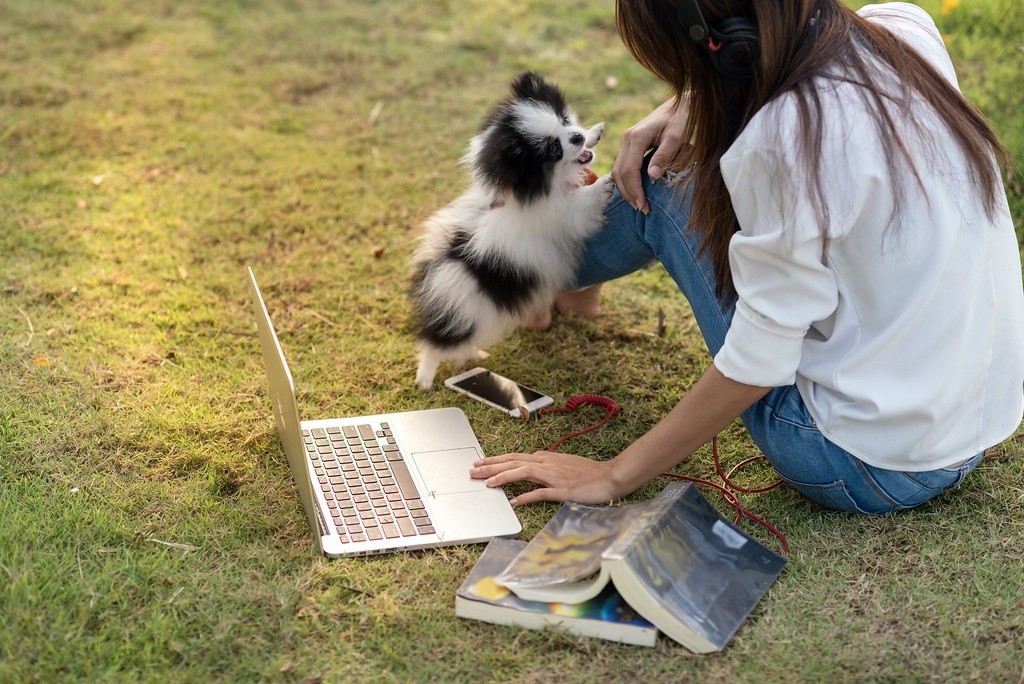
[247,265,525,560]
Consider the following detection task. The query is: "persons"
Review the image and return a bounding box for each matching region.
[471,1,1023,516]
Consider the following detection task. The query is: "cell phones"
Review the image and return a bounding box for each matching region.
[444,367,554,420]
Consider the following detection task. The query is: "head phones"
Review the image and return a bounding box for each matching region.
[680,0,764,83]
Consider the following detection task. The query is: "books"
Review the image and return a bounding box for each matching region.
[494,482,788,654]
[455,537,658,648]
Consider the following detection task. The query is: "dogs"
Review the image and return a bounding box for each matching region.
[403,67,616,392]
